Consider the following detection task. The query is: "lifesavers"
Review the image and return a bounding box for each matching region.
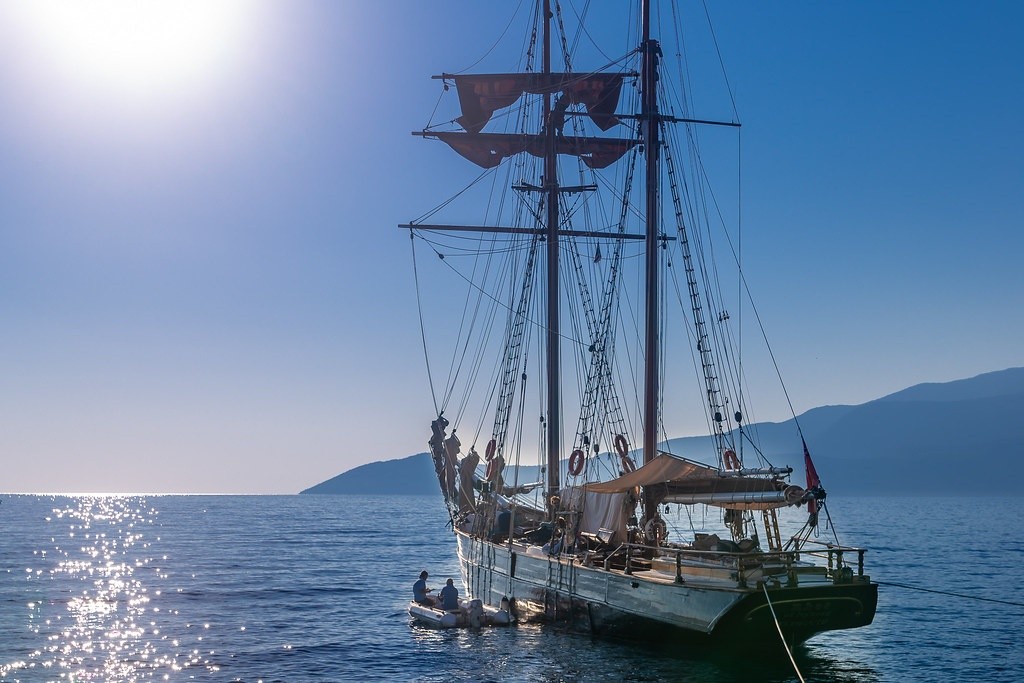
[615,434,629,456]
[484,459,496,481]
[723,450,738,469]
[568,450,584,476]
[645,517,667,541]
[485,439,496,461]
[622,457,636,472]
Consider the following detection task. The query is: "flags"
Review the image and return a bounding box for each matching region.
[802,438,821,513]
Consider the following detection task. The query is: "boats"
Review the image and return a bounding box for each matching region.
[406,598,512,629]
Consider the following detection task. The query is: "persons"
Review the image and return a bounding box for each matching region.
[413,571,436,610]
[441,579,458,609]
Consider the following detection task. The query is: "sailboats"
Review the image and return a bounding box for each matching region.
[399,0,879,657]
